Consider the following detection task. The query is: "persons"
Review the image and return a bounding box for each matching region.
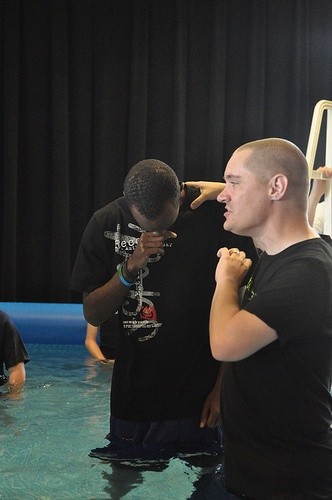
[85,310,116,363]
[182,137,332,500]
[69,159,260,460]
[307,165,332,235]
[0,309,31,401]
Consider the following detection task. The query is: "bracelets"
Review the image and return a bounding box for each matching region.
[122,260,138,280]
[116,261,136,287]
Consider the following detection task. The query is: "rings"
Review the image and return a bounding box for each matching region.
[230,251,240,255]
